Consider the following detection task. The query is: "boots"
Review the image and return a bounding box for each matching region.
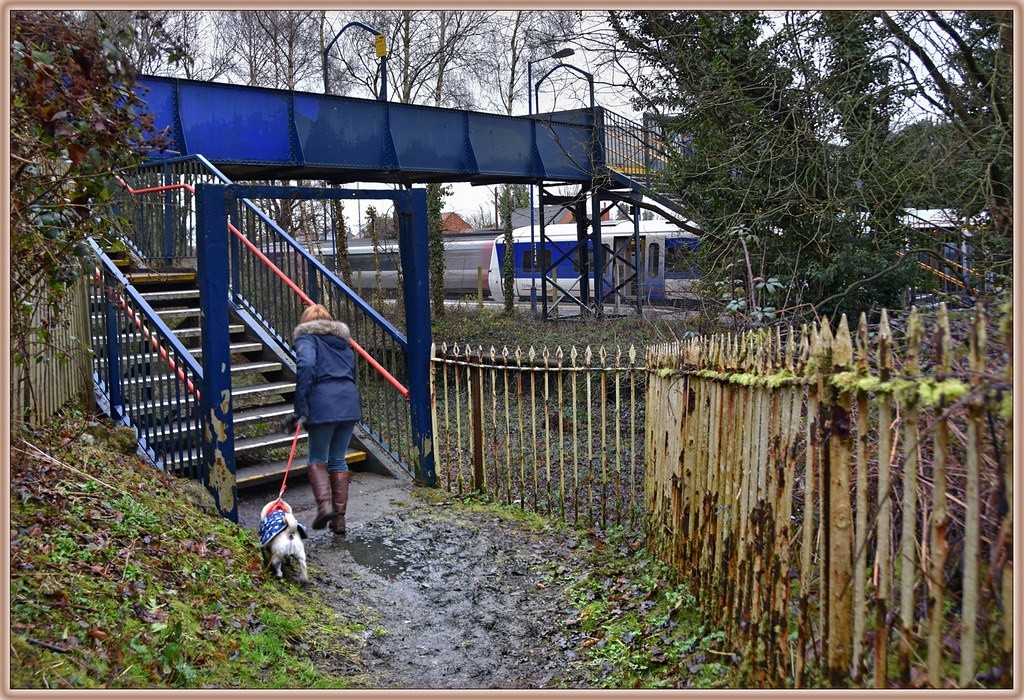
[308,463,335,529]
[328,471,350,535]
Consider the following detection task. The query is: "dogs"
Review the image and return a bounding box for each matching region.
[260,500,310,589]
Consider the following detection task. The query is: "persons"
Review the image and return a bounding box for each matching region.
[294,304,362,534]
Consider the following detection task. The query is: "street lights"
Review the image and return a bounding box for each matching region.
[528,47,576,314]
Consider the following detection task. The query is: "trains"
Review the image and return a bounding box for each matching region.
[487,205,990,306]
[259,237,493,300]
